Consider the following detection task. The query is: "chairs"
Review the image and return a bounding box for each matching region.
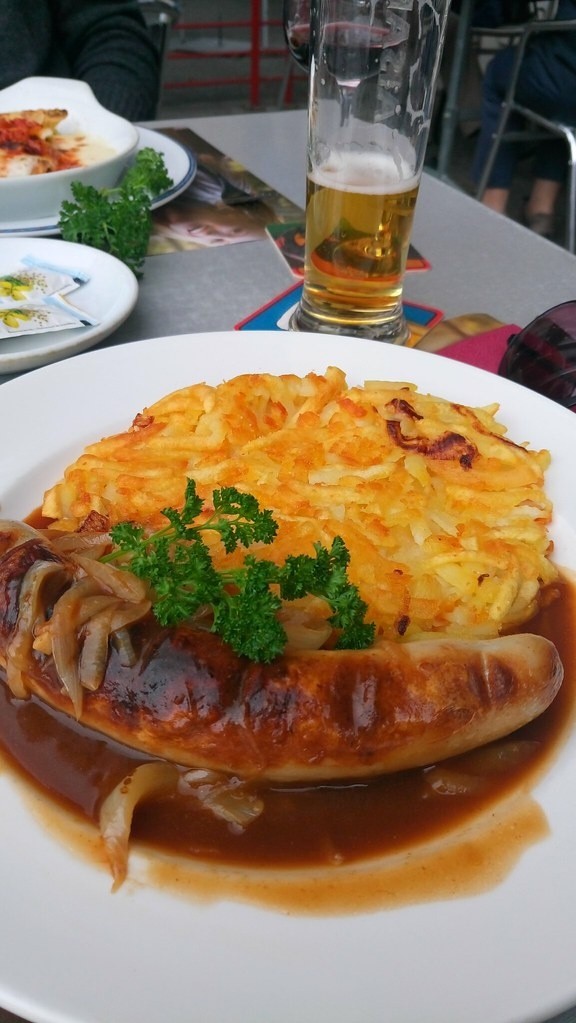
[476,19,576,255]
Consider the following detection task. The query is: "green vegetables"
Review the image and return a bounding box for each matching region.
[54,146,177,283]
[91,476,376,663]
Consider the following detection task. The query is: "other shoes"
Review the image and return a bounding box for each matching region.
[510,200,561,238]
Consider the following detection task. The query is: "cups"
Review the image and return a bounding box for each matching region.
[294,0,451,347]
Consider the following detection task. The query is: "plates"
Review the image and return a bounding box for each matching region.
[0,127,196,237]
[0,331,576,1022]
[1,238,140,373]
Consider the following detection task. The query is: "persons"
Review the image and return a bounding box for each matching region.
[476,33,576,236]
[0,1,162,121]
[145,166,285,251]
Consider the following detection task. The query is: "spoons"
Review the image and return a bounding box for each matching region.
[197,163,263,207]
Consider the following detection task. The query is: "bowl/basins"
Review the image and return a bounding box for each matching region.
[0,76,140,222]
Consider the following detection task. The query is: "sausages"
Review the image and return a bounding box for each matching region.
[0,517,564,783]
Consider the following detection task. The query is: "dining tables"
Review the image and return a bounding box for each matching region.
[0,109,576,385]
